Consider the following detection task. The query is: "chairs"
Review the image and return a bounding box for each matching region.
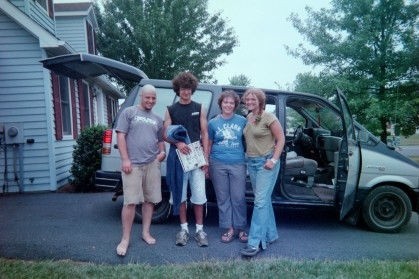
[286,156,318,176]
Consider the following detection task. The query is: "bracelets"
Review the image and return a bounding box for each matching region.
[160,150,166,155]
[271,158,278,164]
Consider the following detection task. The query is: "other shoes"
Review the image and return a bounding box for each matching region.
[195,230,208,247]
[176,229,190,246]
[241,245,262,256]
[141,232,156,245]
[237,228,248,243]
[116,240,130,256]
[220,228,238,243]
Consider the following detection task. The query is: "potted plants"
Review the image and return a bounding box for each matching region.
[69,127,104,192]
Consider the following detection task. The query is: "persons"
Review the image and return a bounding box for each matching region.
[114,84,167,255]
[205,90,250,242]
[239,87,285,256]
[163,71,210,247]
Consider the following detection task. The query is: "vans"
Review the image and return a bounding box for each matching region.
[38,52,419,234]
